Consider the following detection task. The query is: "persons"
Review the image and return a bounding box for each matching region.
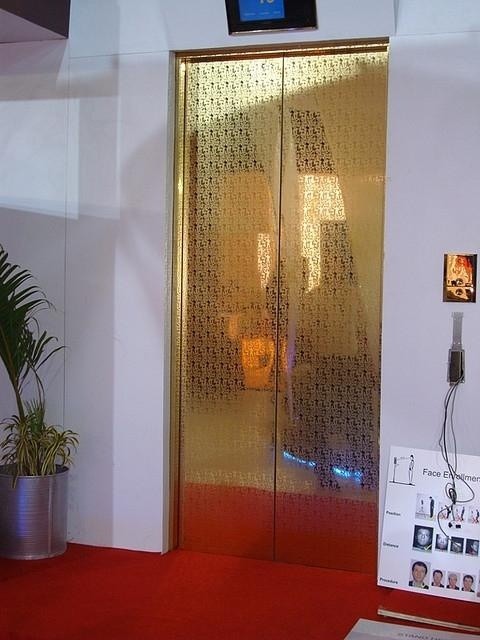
[446,573,460,591]
[428,496,435,519]
[475,508,480,523]
[408,561,430,590]
[413,527,433,552]
[461,574,475,593]
[431,570,445,589]
[444,504,452,519]
[437,533,448,550]
[460,506,465,521]
[468,540,479,556]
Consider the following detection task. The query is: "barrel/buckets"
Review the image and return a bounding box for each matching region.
[0,463,68,560]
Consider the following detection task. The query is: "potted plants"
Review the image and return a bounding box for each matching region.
[0,242,80,562]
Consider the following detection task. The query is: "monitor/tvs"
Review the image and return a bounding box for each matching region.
[225,0,318,35]
